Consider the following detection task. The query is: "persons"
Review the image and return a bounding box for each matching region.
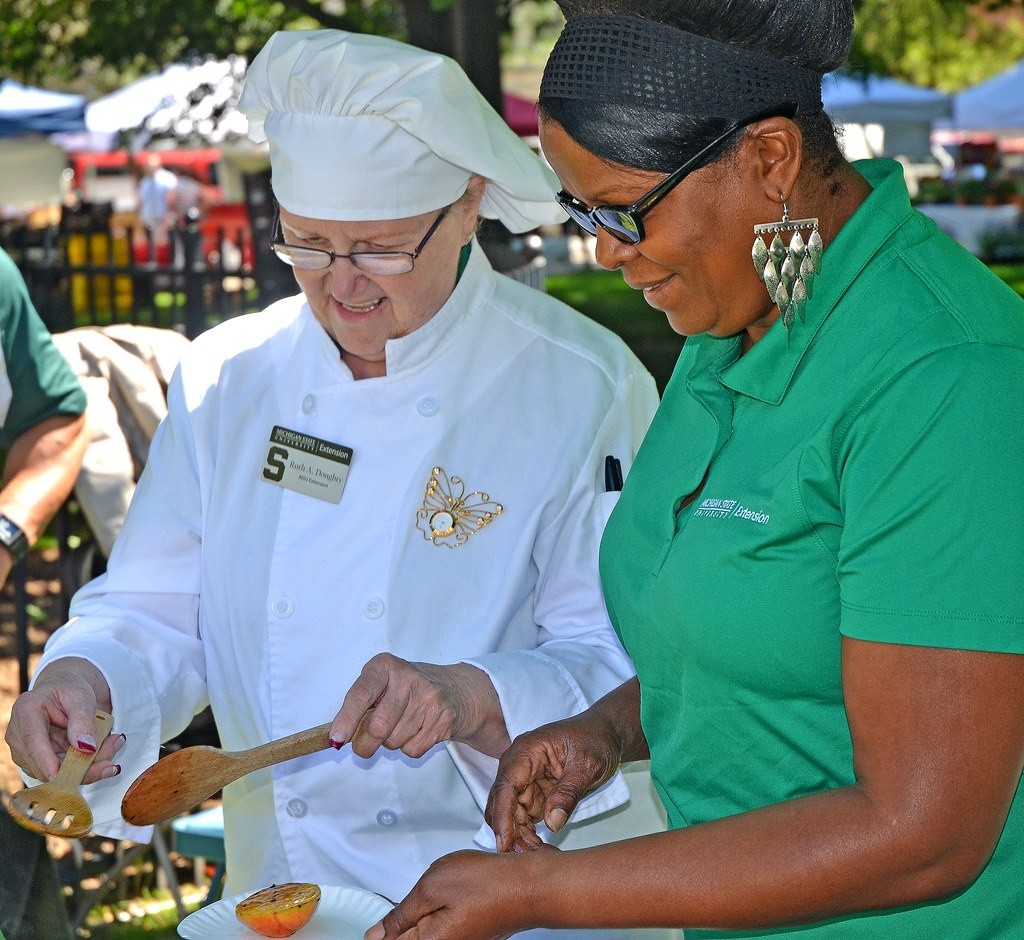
[368,3,1023,940]
[0,24,681,940]
[0,234,89,591]
[125,142,221,276]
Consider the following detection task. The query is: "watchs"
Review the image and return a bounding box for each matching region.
[0,512,31,563]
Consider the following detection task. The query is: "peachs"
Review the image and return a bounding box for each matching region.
[234,882,320,938]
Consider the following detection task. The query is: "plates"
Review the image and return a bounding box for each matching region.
[176,884,399,940]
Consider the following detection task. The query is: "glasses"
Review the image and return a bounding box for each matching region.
[266,193,456,276]
[555,100,801,247]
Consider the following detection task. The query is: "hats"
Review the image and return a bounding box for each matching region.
[243,26,570,236]
